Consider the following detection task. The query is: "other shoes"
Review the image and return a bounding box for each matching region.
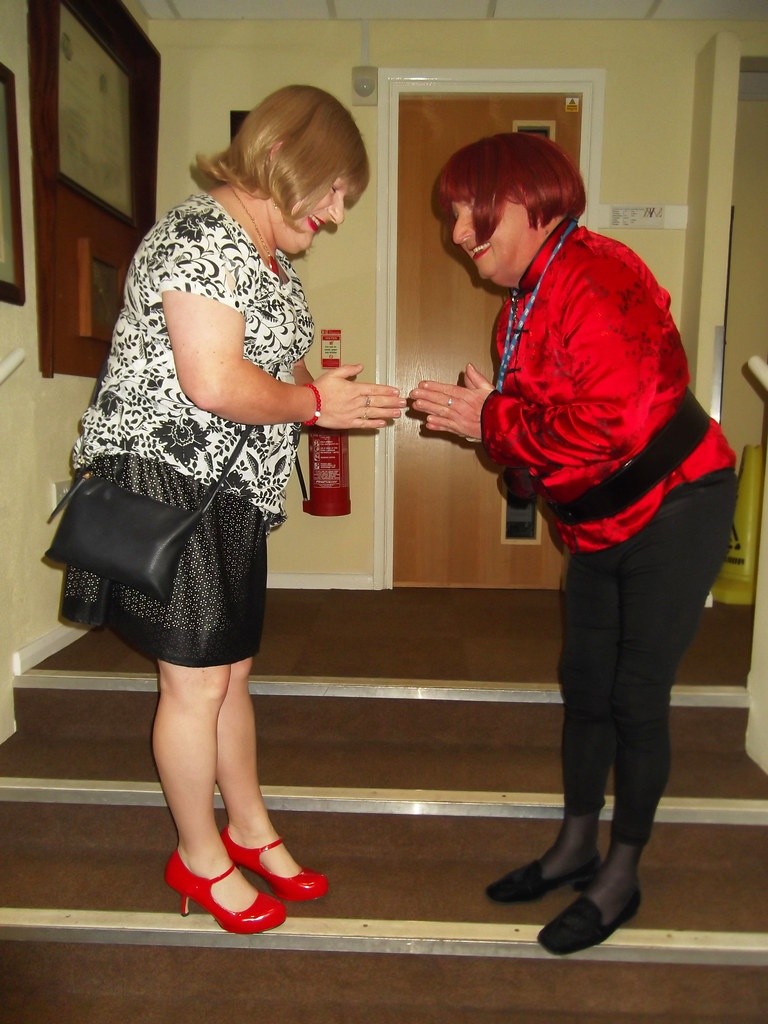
[537,886,641,955]
[485,851,601,903]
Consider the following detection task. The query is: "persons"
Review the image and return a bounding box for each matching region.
[409,134,738,958]
[59,83,407,934]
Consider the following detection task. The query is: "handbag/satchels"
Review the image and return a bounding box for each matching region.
[44,472,204,604]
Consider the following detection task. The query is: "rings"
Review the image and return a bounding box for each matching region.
[363,408,367,420]
[448,398,451,406]
[366,395,370,406]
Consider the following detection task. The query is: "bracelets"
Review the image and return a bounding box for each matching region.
[304,384,321,426]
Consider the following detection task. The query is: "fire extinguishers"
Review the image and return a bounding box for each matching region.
[292,424,352,516]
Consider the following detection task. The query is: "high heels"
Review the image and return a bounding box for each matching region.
[164,848,287,935]
[220,824,329,902]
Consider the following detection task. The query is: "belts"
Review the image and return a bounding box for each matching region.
[545,388,709,526]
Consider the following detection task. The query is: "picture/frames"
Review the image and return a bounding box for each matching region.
[0,61,26,306]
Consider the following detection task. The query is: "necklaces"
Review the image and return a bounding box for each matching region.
[228,183,279,276]
